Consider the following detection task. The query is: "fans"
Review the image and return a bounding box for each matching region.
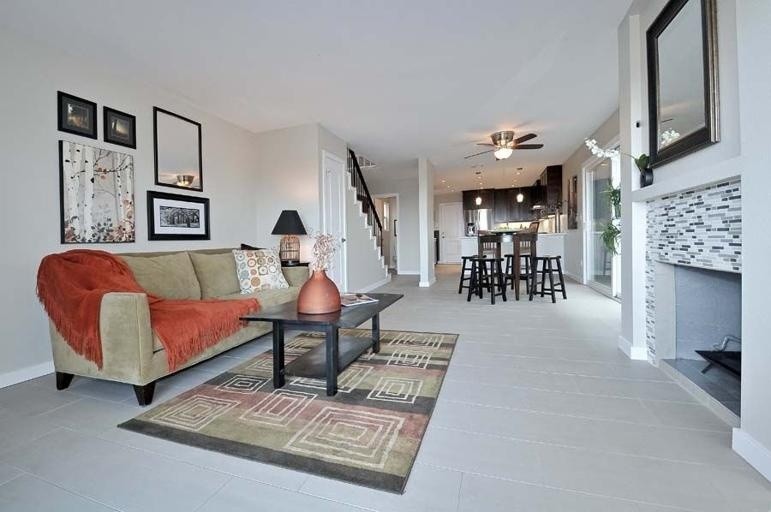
[463,131,545,161]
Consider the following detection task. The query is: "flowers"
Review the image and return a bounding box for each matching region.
[307,230,342,272]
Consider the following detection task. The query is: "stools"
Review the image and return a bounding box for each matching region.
[504,254,537,295]
[458,254,490,295]
[528,256,567,305]
[467,257,507,305]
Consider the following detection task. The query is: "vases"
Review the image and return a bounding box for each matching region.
[297,269,342,315]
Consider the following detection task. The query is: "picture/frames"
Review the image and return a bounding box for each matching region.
[152,105,203,193]
[56,90,98,141]
[102,105,138,150]
[145,190,208,238]
[58,139,136,244]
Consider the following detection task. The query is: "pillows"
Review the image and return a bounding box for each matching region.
[114,251,202,301]
[188,250,241,301]
[232,247,290,296]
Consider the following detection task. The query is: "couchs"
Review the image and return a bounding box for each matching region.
[34,247,311,408]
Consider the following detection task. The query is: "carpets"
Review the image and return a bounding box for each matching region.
[113,325,461,497]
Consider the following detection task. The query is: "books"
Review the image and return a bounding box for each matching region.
[340,293,379,307]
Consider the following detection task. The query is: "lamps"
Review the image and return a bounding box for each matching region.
[494,148,513,160]
[270,209,308,268]
